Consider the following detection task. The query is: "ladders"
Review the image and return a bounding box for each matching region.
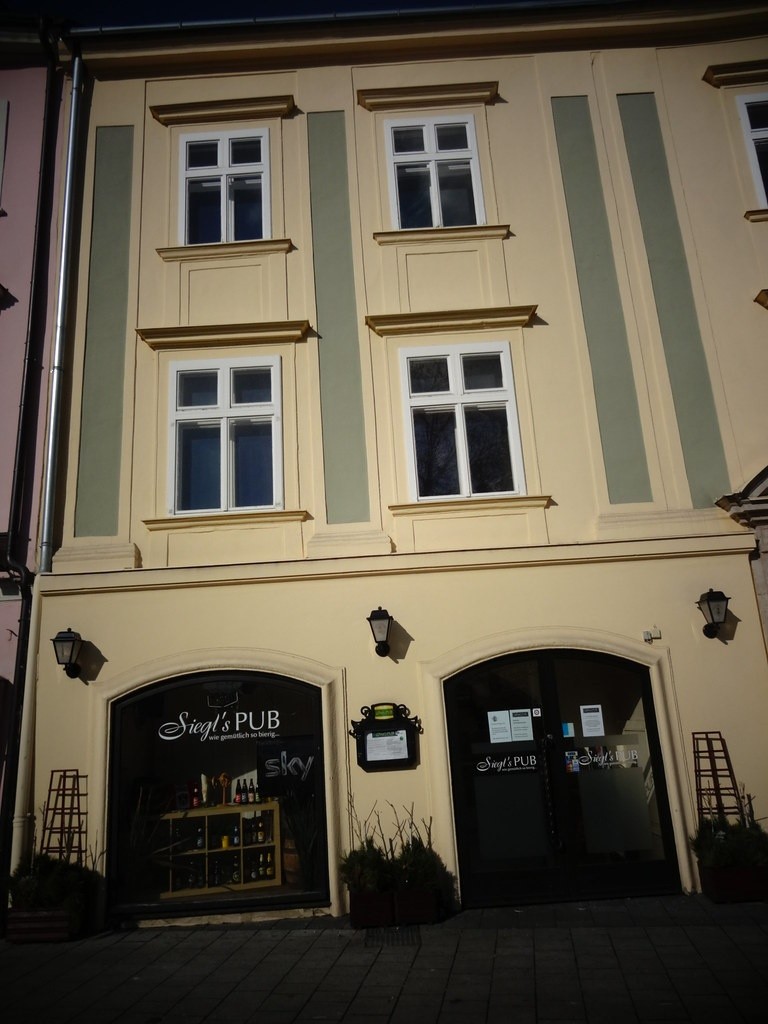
[692,731,747,832]
[39,768,81,868]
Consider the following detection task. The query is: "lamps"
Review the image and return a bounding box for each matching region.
[366,605,393,657]
[49,626,84,678]
[696,587,733,639]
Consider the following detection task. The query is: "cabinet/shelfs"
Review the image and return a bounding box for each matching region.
[132,799,282,899]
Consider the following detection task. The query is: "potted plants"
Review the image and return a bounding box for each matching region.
[391,835,457,927]
[0,853,80,944]
[336,837,396,932]
[692,815,768,903]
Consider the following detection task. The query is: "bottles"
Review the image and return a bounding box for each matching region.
[248,779,255,803]
[192,784,199,808]
[257,821,265,843]
[235,779,241,803]
[213,861,219,886]
[259,854,266,880]
[265,853,273,879]
[255,787,261,803]
[231,856,241,883]
[233,826,239,846]
[241,779,248,804]
